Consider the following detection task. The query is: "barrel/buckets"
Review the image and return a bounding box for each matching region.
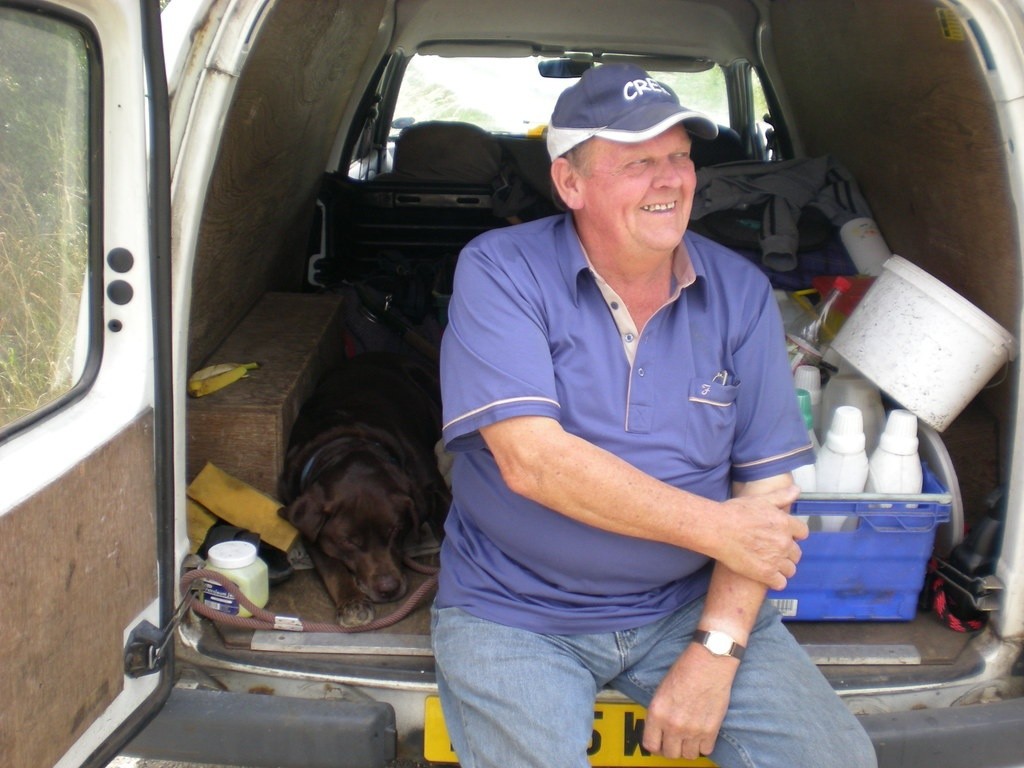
[832,255,1020,432]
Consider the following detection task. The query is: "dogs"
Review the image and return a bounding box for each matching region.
[275,347,431,629]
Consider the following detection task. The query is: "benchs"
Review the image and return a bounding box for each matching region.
[187,287,349,501]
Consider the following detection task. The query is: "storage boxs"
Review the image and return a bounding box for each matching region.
[764,462,951,621]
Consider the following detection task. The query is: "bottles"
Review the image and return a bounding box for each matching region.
[766,217,924,530]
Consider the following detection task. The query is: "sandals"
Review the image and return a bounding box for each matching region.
[196,522,296,584]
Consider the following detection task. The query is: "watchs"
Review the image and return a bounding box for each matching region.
[692,628,747,661]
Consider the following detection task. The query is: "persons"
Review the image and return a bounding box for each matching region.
[430,64,879,768]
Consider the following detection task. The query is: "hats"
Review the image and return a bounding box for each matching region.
[546,62,719,163]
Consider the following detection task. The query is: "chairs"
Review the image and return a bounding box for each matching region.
[691,126,748,169]
[390,122,500,191]
